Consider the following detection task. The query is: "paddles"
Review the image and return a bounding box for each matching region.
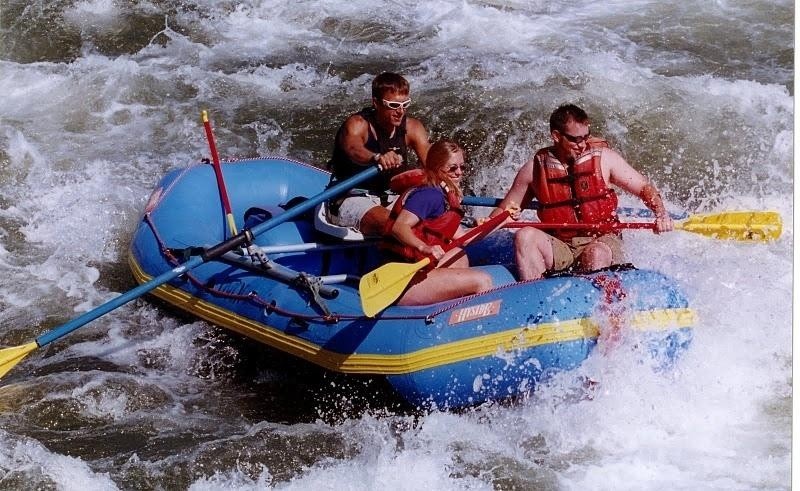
[477,211,780,244]
[0,153,403,379]
[360,202,521,318]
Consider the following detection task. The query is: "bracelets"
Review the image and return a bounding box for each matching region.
[371,153,380,165]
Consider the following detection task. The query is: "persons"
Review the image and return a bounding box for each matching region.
[475,104,674,282]
[321,70,431,236]
[381,138,492,307]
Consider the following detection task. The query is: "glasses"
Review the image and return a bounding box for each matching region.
[382,97,412,111]
[563,131,591,143]
[448,165,467,172]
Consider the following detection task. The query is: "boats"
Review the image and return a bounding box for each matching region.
[126,156,697,416]
